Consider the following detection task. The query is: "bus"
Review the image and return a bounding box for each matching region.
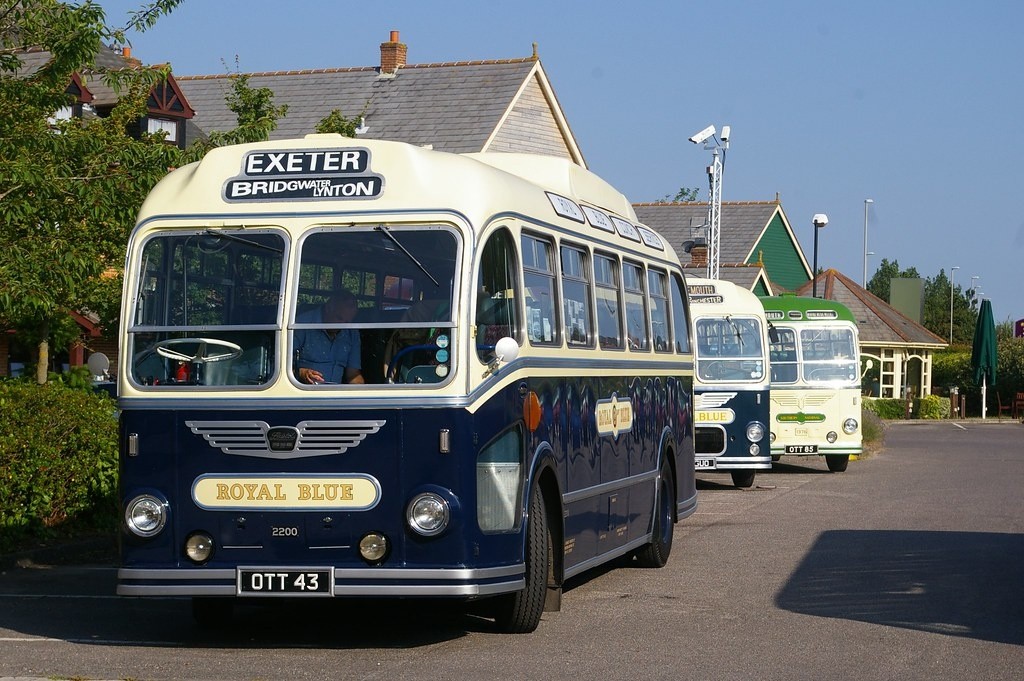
[86,132,700,636]
[681,276,779,487]
[758,293,874,475]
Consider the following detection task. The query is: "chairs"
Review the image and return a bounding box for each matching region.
[384,296,451,384]
[492,296,535,345]
[425,292,497,366]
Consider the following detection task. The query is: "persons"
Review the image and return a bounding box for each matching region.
[293,288,366,386]
[868,375,880,397]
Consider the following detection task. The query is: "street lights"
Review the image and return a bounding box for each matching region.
[950,266,960,344]
[811,213,830,298]
[973,285,985,315]
[863,198,874,291]
[971,276,979,291]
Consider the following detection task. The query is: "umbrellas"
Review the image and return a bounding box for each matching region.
[972,299,998,419]
